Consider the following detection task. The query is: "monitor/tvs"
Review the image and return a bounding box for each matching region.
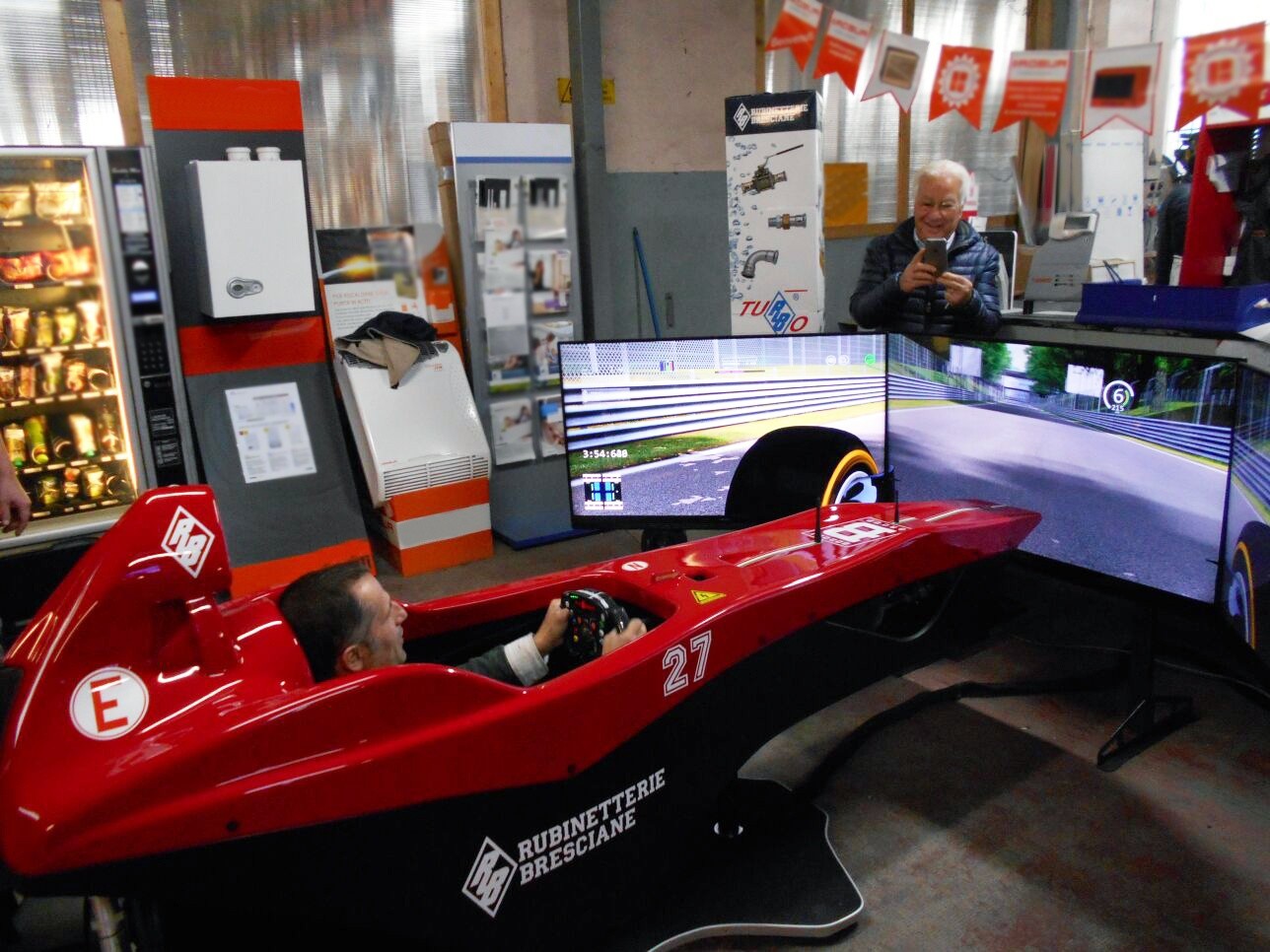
[890,330,1251,608]
[557,335,887,532]
[1213,360,1270,672]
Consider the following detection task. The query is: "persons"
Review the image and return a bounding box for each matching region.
[1154,173,1193,286]
[849,160,1003,361]
[0,432,32,537]
[275,560,648,689]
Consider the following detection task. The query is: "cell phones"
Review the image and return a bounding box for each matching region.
[925,237,947,282]
